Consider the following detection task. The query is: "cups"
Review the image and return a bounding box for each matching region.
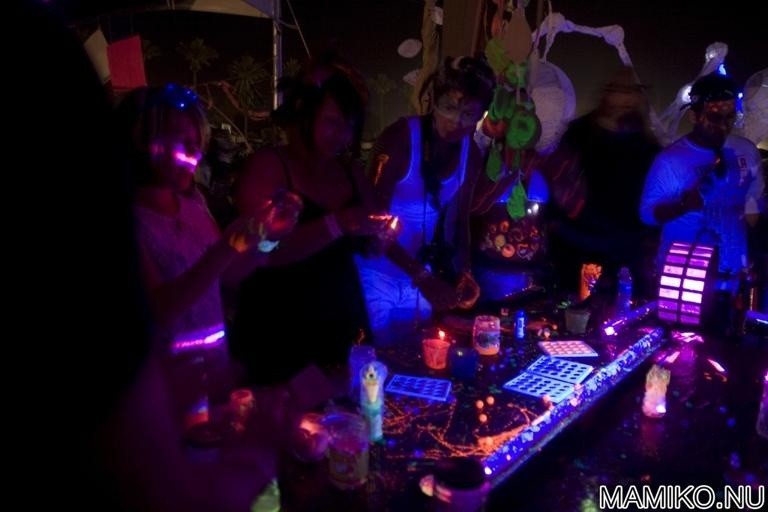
[472,315,501,355]
[325,413,369,490]
[420,340,449,370]
[565,310,591,337]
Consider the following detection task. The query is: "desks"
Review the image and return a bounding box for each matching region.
[268,284,671,512]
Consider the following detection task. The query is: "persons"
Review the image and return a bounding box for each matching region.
[1,0,767,512]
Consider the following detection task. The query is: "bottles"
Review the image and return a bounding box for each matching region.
[514,309,528,342]
[615,267,633,319]
[350,346,375,407]
